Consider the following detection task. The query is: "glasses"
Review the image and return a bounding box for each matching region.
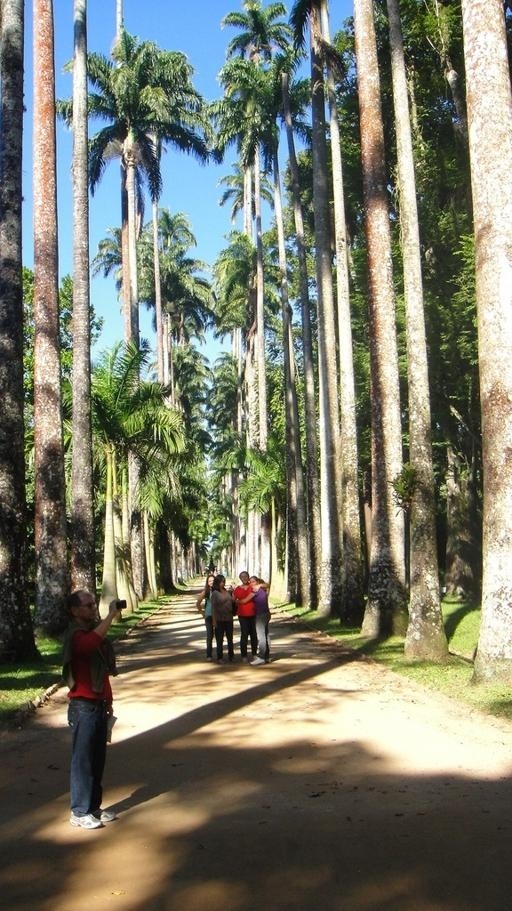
[76,601,96,609]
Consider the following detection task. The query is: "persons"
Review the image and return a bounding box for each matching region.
[197,571,271,666]
[61,590,122,829]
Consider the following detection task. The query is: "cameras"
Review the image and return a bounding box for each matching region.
[116,600,126,609]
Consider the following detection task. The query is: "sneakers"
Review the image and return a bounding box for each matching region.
[207,654,270,666]
[70,808,116,829]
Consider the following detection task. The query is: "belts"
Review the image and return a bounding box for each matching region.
[76,695,111,710]
